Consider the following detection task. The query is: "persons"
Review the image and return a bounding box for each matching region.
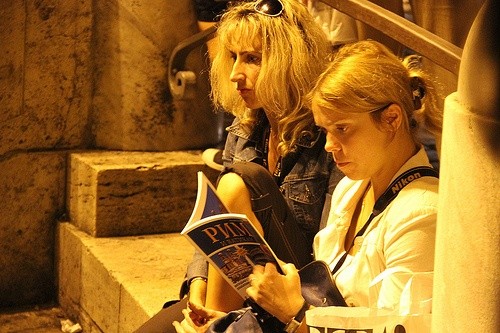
[196,0,228,62]
[132,0,341,333]
[245,40,443,333]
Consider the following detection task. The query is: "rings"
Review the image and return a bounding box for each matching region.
[250,277,255,283]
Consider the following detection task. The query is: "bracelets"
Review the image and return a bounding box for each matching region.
[190,278,207,282]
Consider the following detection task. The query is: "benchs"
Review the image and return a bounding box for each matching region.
[52,150,219,333]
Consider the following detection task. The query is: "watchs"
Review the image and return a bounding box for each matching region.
[284,300,310,333]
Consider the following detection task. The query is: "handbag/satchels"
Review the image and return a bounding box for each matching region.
[199,261,347,333]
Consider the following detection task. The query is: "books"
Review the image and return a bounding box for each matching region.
[180,170,286,300]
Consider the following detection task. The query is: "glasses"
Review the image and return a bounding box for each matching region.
[253,0,287,18]
